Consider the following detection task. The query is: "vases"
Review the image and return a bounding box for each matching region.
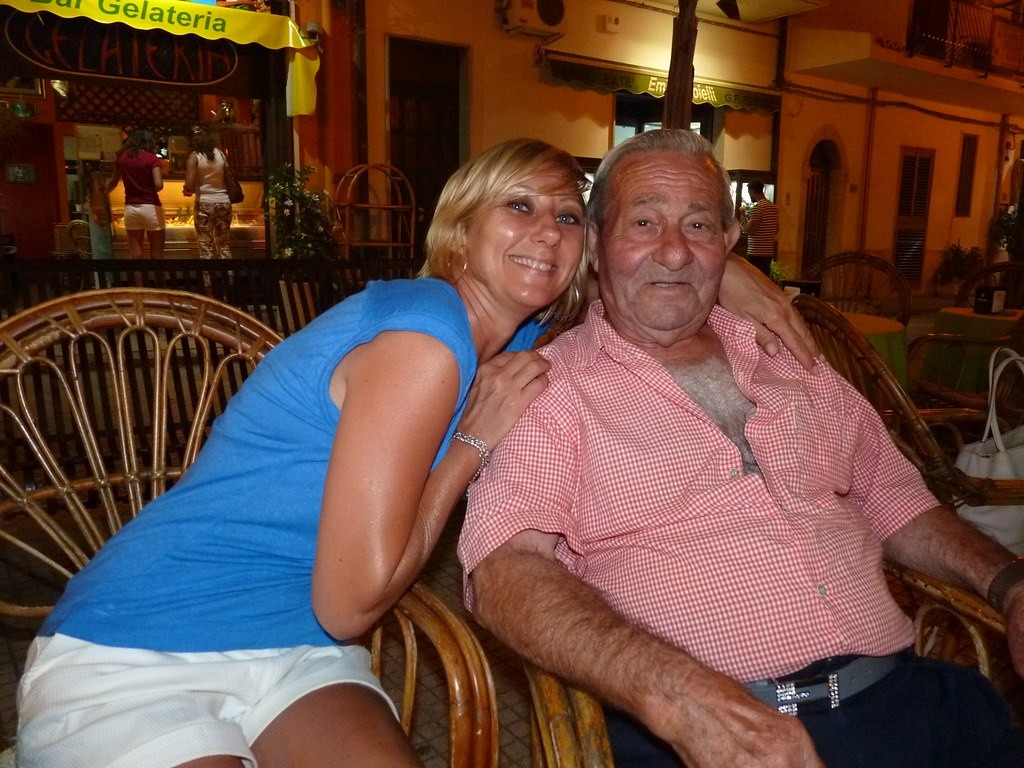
[277,280,319,332]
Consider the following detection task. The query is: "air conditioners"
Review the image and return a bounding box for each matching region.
[502,0,566,37]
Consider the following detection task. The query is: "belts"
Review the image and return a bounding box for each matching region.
[747,649,898,720]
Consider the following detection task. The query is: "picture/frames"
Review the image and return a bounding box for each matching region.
[4,164,36,183]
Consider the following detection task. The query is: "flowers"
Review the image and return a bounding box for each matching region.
[258,162,340,282]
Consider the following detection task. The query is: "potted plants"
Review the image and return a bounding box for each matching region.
[992,209,1024,285]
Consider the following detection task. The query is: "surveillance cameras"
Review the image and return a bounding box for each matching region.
[307,23,320,38]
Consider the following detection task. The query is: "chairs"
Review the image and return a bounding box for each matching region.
[523,560,1024,768]
[791,294,1024,518]
[800,251,912,328]
[67,220,93,259]
[954,261,1024,307]
[0,289,498,768]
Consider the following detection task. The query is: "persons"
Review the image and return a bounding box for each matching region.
[456,130,1024,768]
[740,179,779,277]
[183,131,233,298]
[18,140,590,768]
[106,127,165,288]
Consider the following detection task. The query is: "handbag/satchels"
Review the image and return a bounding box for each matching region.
[949,346,1024,558]
[216,148,244,204]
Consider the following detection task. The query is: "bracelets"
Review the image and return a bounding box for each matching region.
[740,220,746,226]
[987,559,1024,612]
[450,431,489,481]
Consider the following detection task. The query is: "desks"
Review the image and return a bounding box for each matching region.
[922,307,1024,394]
[839,312,907,410]
